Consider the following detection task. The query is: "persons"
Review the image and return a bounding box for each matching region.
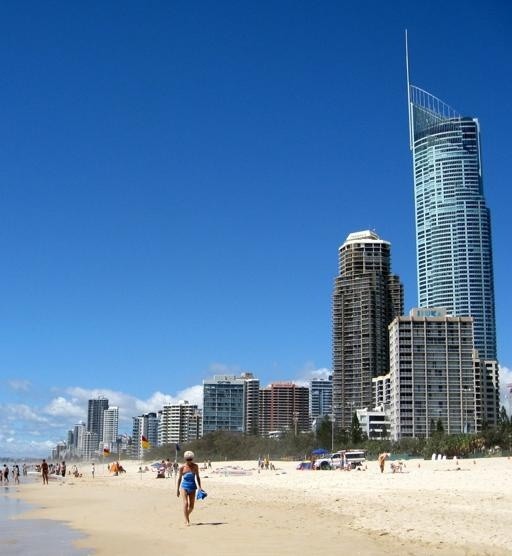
[339,452,345,469]
[380,454,386,473]
[36,459,66,485]
[259,455,275,470]
[73,465,79,479]
[0,463,27,484]
[204,458,211,468]
[91,463,95,478]
[313,448,328,463]
[108,461,127,474]
[160,458,179,477]
[176,450,201,527]
[311,454,316,470]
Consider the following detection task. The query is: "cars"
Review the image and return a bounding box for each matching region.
[314,452,366,470]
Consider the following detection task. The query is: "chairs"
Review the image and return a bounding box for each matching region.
[429,453,460,472]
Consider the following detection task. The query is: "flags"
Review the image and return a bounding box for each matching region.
[176,445,180,450]
[104,448,111,456]
[142,436,149,449]
